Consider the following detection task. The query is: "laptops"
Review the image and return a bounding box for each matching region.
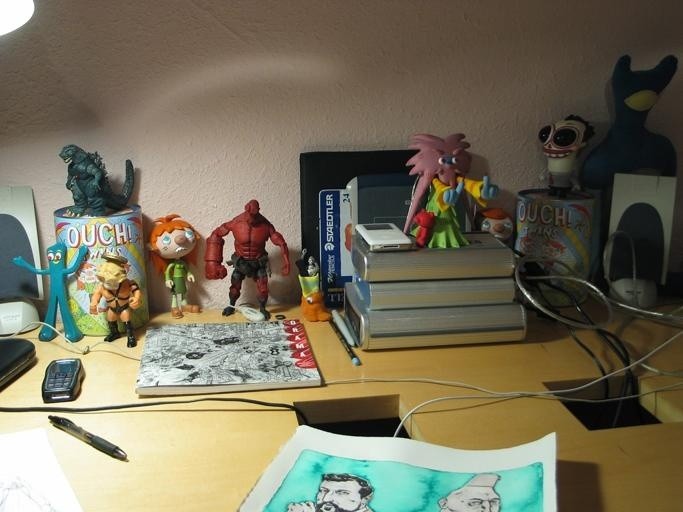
[0,340,36,389]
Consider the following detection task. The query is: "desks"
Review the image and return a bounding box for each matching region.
[1,287,680,510]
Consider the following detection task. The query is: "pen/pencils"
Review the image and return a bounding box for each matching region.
[48,415,126,459]
[328,309,362,366]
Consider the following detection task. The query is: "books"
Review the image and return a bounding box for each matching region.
[135,320,321,395]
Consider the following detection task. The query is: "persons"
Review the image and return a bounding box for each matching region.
[150,215,200,318]
[90,255,144,347]
[204,200,290,319]
[438,472,501,512]
[287,474,374,512]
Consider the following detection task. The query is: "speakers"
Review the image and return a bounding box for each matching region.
[602,171,678,312]
[0,185,45,336]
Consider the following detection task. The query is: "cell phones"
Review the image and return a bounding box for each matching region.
[355,222,412,253]
[41,358,84,403]
